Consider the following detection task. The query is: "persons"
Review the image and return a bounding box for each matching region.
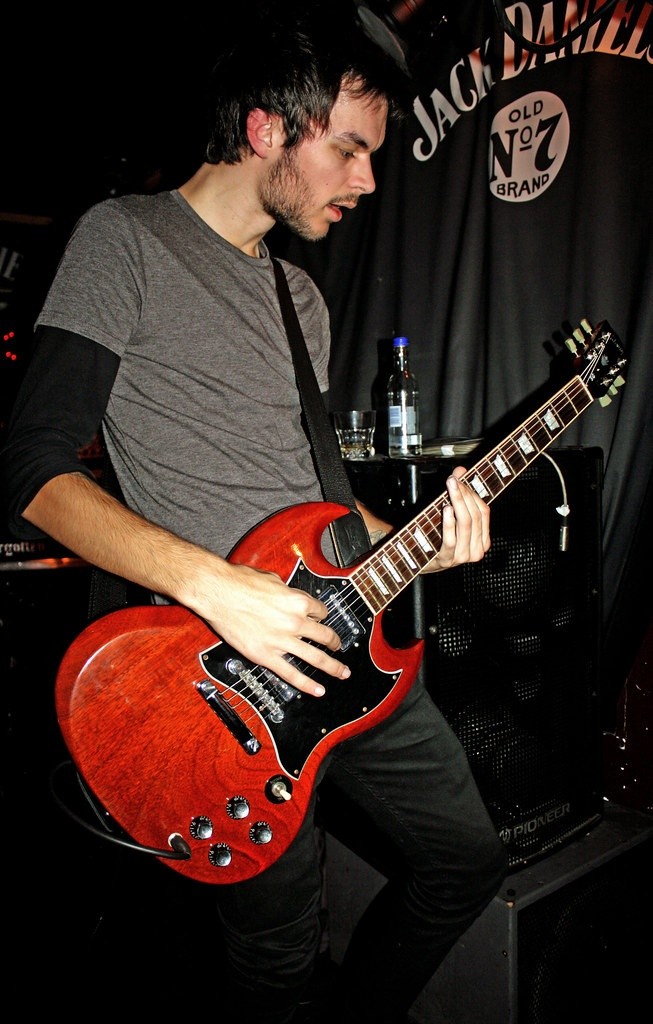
[0,2,509,1020]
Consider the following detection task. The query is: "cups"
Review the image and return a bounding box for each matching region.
[332,407,378,460]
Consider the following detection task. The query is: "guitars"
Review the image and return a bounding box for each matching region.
[50,319,632,887]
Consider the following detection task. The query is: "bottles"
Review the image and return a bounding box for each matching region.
[385,335,423,457]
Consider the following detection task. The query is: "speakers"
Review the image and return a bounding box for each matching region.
[323,816,652,1023]
[320,445,611,876]
